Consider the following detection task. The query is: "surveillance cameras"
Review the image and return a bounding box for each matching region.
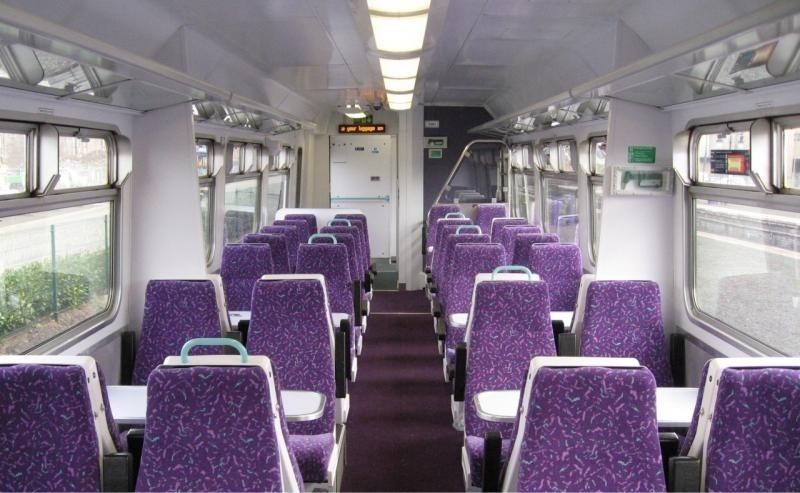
[373,103,381,110]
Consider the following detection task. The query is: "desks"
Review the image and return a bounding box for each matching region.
[105,383,328,425]
[472,387,700,428]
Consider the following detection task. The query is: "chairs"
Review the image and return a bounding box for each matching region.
[245,274,351,491]
[484,356,666,491]
[119,273,226,452]
[126,354,308,492]
[425,199,584,383]
[672,355,799,490]
[459,272,559,492]
[567,273,679,453]
[218,209,377,383]
[2,354,131,491]
